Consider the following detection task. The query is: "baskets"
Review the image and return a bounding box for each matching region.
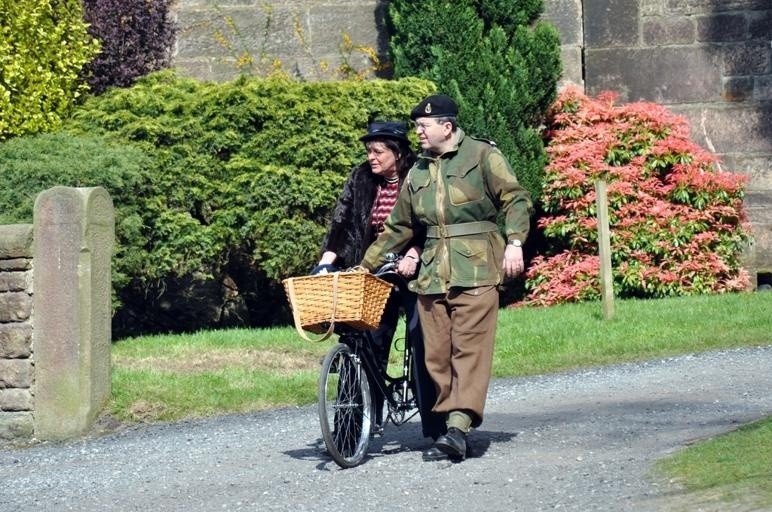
[281,271,395,343]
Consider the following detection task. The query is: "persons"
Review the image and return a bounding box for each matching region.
[358,96,533,462]
[318,120,446,456]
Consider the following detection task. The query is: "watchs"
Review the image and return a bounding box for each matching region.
[508,239,522,246]
[405,253,420,263]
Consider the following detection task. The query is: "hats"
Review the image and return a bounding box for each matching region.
[359,122,412,144]
[410,95,458,121]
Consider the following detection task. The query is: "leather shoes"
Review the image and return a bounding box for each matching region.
[318,437,358,455]
[423,427,466,461]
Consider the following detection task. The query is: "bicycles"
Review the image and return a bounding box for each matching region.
[281,252,417,469]
